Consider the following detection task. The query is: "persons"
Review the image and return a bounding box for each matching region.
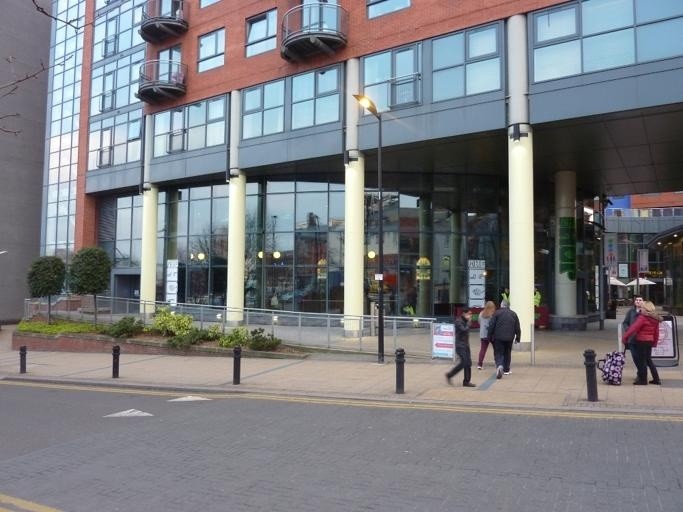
[499,287,510,309]
[623,301,661,385]
[487,299,522,379]
[445,309,476,387]
[534,287,541,326]
[478,301,498,369]
[623,296,645,384]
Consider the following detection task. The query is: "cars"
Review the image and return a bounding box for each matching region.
[199,272,373,309]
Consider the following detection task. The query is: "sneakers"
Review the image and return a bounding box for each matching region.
[463,381,477,387]
[504,368,513,375]
[650,380,662,385]
[496,364,504,378]
[477,366,482,369]
[633,381,648,385]
[445,373,452,386]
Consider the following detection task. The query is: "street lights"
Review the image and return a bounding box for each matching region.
[350,92,387,366]
[183,248,376,301]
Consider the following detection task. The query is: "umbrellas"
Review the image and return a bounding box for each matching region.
[610,277,626,286]
[626,278,656,285]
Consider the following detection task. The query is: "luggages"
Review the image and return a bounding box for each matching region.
[600,349,625,386]
